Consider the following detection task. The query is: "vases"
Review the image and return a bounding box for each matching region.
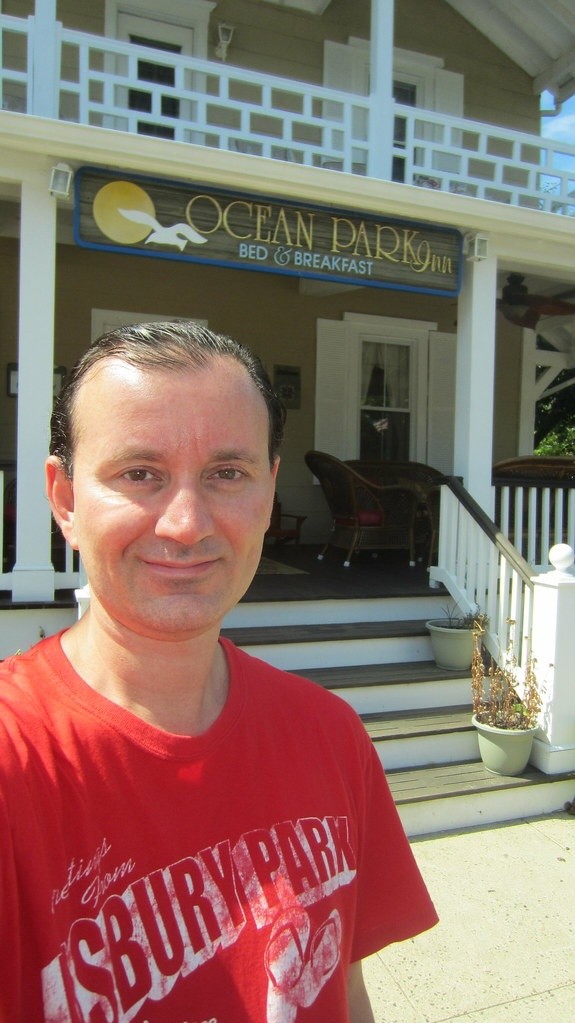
[472,711,540,776]
[426,619,482,671]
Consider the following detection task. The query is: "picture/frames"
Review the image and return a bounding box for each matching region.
[7,362,18,397]
[54,366,67,399]
[273,364,300,409]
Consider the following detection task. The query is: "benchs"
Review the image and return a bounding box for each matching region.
[426,456,575,572]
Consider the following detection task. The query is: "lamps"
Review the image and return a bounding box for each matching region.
[49,163,74,201]
[214,21,236,62]
[465,230,488,260]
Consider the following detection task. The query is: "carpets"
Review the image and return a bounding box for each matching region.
[255,556,309,575]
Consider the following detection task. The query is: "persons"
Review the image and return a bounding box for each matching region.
[0,318,439,1023]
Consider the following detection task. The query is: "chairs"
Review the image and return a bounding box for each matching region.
[304,450,419,567]
[344,460,446,544]
